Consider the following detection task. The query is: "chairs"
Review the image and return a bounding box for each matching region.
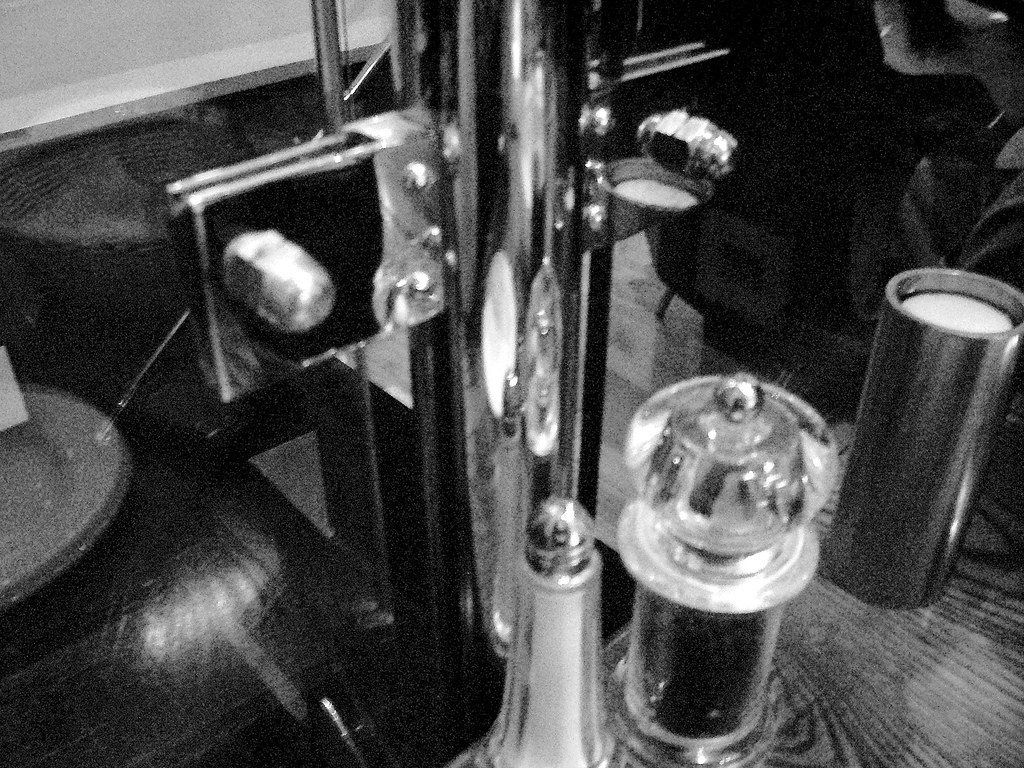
[646,197,838,334]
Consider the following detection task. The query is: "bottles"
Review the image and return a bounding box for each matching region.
[469,494,614,768]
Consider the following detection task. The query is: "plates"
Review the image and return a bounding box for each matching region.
[0,382,132,612]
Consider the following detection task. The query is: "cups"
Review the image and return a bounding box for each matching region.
[821,267,1024,610]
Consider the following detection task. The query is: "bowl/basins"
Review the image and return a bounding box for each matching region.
[595,159,714,221]
